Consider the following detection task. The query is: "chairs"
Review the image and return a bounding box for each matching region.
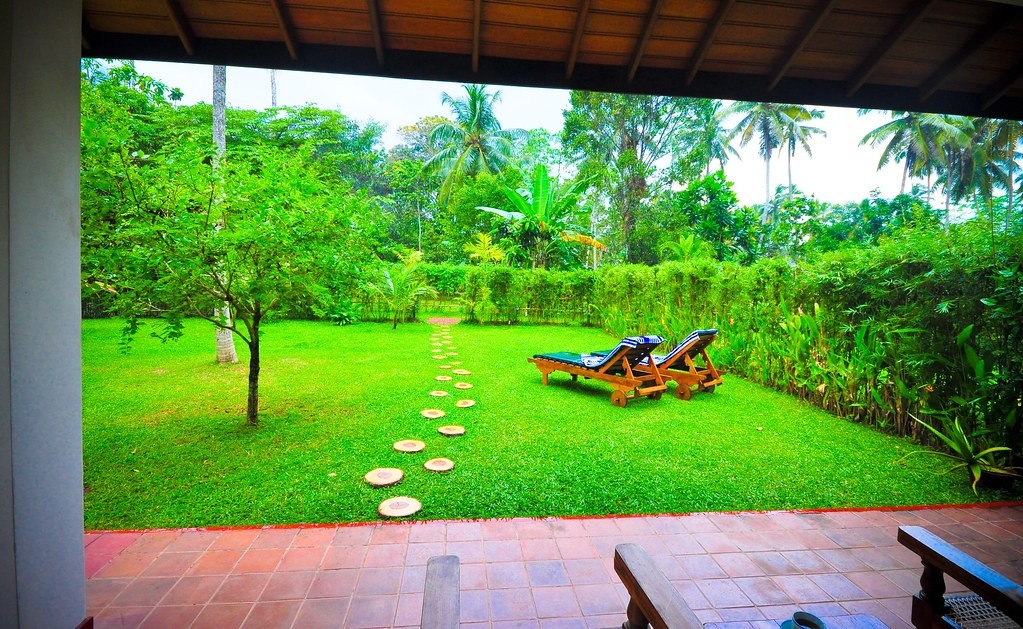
[614,524,1023,629]
[527,334,668,407]
[592,329,727,401]
